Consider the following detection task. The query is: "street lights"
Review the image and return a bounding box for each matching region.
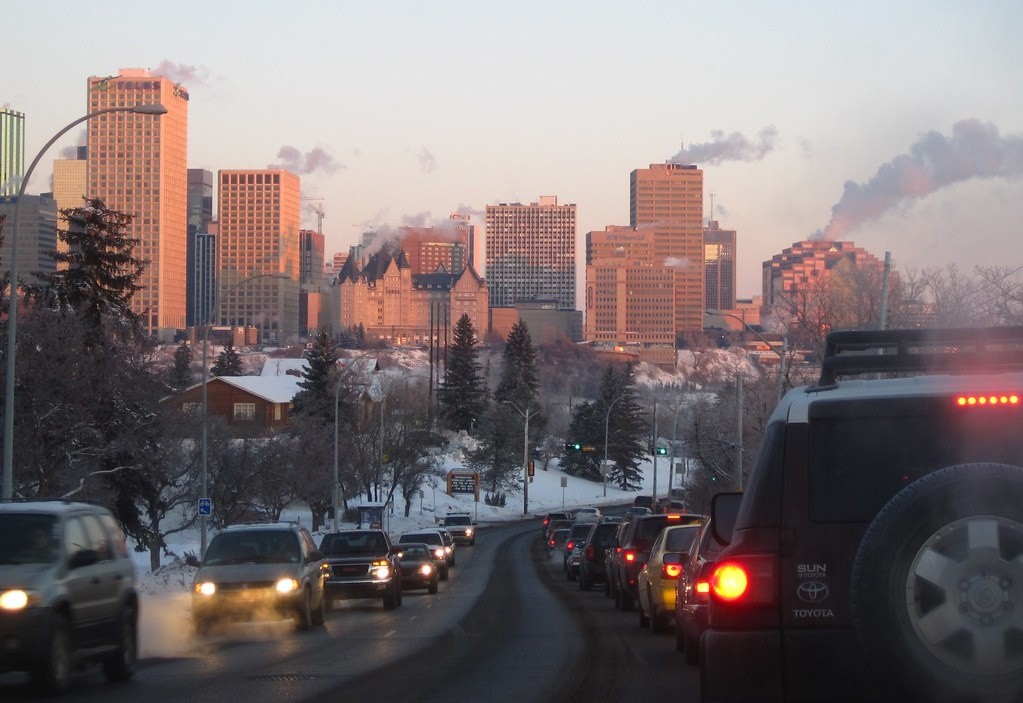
[377,374,427,525]
[503,400,548,512]
[625,383,680,511]
[333,348,394,531]
[704,308,790,407]
[602,392,642,495]
[200,273,289,568]
[0,101,178,501]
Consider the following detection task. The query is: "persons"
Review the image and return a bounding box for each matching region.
[31,522,55,562]
[366,537,377,551]
[412,549,420,558]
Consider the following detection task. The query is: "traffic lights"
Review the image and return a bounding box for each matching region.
[652,447,668,457]
[565,442,581,451]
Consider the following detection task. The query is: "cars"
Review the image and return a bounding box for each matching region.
[391,542,439,595]
[317,527,408,609]
[546,497,731,665]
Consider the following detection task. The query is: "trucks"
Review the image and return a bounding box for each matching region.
[444,510,477,546]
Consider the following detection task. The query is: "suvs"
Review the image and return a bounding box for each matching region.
[0,502,140,703]
[693,321,1022,703]
[185,519,327,634]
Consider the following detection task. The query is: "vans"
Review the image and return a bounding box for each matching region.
[395,532,452,580]
[419,527,455,570]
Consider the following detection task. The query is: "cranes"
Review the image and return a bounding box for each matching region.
[308,201,328,233]
